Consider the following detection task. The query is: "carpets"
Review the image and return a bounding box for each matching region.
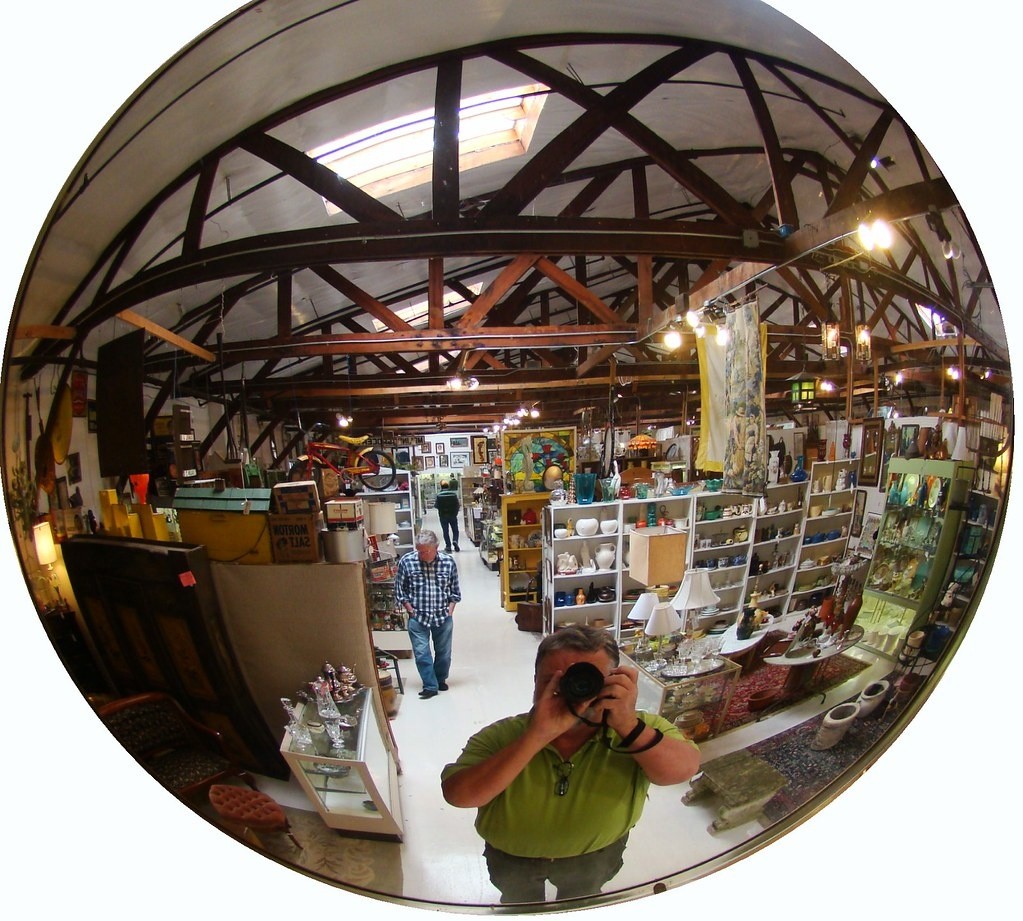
[748,668,929,828]
[700,651,870,740]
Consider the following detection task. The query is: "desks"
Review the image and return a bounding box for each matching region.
[683,752,792,835]
[715,614,774,666]
[764,624,866,701]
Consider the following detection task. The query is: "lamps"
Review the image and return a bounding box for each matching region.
[645,601,684,669]
[670,566,721,621]
[628,593,660,654]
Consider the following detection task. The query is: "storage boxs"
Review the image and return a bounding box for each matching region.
[269,509,326,563]
[326,494,363,524]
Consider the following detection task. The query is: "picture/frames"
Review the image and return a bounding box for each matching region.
[854,417,885,487]
[412,436,487,472]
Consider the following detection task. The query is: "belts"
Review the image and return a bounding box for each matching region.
[486,842,605,863]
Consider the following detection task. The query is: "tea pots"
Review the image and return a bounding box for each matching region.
[338,660,358,684]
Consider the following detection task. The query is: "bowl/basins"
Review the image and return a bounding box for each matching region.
[555,529,568,538]
[954,566,976,583]
[600,520,617,534]
[576,518,599,536]
[674,518,688,527]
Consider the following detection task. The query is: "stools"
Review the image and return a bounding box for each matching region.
[209,785,304,863]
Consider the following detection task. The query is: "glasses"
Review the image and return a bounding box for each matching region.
[553,762,572,796]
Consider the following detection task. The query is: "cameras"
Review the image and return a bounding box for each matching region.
[558,660,607,711]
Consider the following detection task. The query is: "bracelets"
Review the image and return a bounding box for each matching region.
[407,608,415,614]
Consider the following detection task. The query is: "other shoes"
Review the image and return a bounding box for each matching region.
[438,682,448,691]
[418,689,438,697]
[452,541,460,552]
[445,547,452,553]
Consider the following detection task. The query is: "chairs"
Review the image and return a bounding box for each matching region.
[97,696,262,809]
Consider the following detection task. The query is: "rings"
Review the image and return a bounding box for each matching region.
[553,692,559,695]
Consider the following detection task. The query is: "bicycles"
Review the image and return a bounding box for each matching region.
[287,422,397,491]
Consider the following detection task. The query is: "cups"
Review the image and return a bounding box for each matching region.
[637,484,648,499]
[572,473,597,505]
[597,477,619,503]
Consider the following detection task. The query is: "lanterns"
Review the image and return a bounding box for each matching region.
[785,371,821,414]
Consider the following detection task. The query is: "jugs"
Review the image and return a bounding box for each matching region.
[311,675,341,718]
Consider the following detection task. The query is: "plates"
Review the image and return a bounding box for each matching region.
[702,608,720,617]
[335,714,357,727]
[707,625,731,634]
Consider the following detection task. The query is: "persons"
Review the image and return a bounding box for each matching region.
[434,483,460,552]
[395,530,462,696]
[448,473,458,492]
[440,624,701,903]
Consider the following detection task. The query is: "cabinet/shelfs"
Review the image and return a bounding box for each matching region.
[846,457,974,662]
[280,686,407,844]
[365,470,858,656]
[618,645,743,747]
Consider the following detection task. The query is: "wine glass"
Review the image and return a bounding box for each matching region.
[677,642,689,676]
[708,638,726,668]
[324,721,345,748]
[690,642,705,675]
